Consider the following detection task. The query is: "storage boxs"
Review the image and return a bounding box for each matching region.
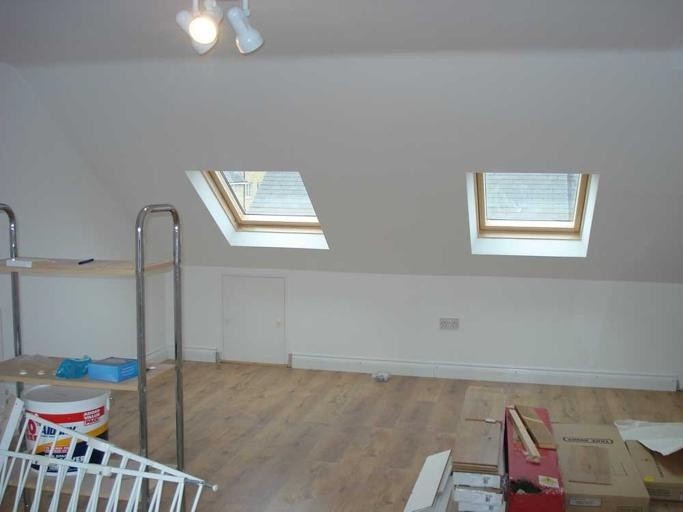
[447,382,682,511]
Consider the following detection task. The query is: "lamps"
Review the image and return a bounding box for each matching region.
[173,0,262,55]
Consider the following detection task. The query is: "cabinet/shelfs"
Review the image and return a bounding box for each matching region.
[0,202,185,512]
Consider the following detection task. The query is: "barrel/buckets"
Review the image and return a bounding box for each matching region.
[20,386,111,475]
